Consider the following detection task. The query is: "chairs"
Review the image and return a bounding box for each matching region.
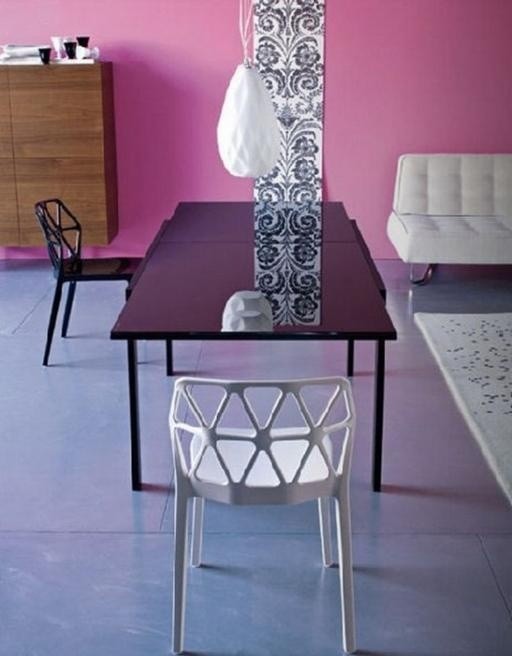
[31,199,174,377]
[168,376,357,655]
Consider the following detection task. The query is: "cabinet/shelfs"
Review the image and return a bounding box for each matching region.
[0,64,119,248]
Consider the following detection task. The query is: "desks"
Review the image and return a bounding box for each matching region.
[112,201,397,491]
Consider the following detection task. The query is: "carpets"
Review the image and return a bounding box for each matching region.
[414,312,511,504]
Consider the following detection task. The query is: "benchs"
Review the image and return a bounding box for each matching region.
[387,153,512,285]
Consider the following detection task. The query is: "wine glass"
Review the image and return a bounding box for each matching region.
[51,35,65,59]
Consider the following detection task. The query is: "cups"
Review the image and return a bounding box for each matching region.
[37,47,53,64]
[64,41,78,59]
[76,36,90,47]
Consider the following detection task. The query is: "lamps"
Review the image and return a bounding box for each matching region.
[215,0,283,179]
